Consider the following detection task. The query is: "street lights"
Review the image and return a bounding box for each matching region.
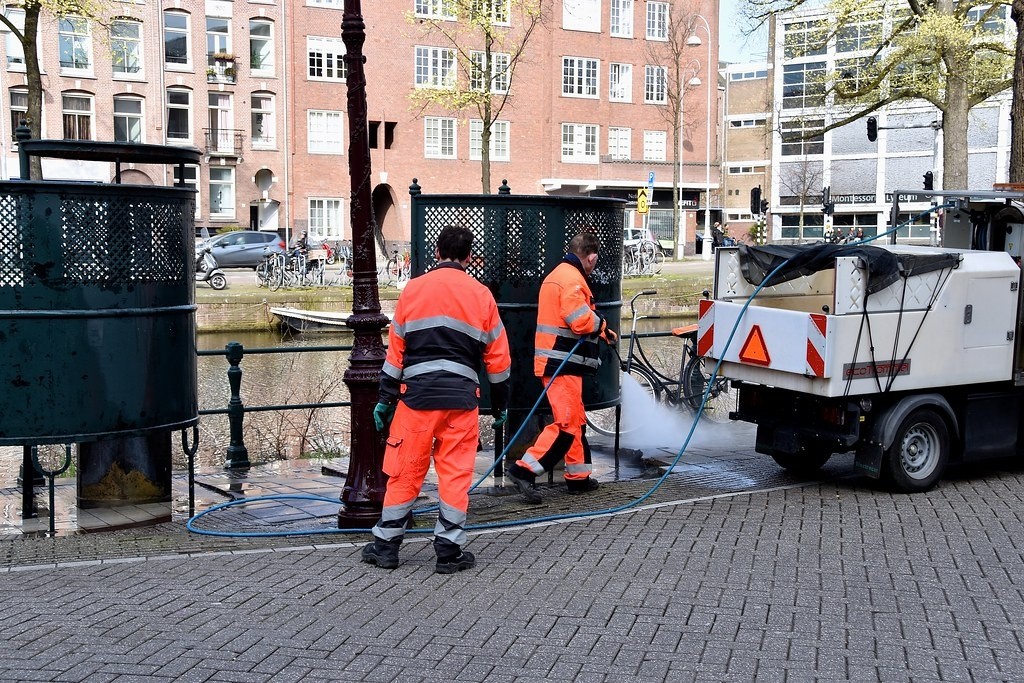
[686,15,714,261]
[677,58,702,260]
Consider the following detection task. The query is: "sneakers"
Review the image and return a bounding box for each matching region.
[436,551,475,574]
[362,543,399,569]
[565,477,599,495]
[505,464,542,504]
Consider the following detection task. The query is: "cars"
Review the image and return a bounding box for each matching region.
[623,227,655,246]
[696,232,704,254]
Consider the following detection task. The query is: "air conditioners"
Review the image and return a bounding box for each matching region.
[602,154,612,164]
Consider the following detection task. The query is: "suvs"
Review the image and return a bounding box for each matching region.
[196,230,286,270]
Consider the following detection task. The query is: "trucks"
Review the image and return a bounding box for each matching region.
[698,187,1024,493]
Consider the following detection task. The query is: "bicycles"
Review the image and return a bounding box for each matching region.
[583,291,739,436]
[624,235,667,273]
[319,237,353,265]
[386,243,412,286]
[255,247,288,291]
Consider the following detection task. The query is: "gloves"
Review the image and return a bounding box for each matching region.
[373,402,396,431]
[492,410,506,429]
[590,310,606,341]
[599,329,618,344]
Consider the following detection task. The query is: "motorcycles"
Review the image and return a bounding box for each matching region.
[288,240,309,274]
[195,238,229,289]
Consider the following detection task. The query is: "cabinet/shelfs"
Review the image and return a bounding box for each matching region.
[713,246,835,317]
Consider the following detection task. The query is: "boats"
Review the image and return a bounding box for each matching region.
[269,306,395,332]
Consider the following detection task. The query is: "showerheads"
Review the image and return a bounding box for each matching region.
[615,350,628,373]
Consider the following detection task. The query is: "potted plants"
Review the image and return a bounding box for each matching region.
[224,54,236,68]
[212,52,225,67]
[224,67,237,82]
[206,68,218,83]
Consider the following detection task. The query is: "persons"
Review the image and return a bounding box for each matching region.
[505,233,618,506]
[361,226,510,574]
[712,222,736,253]
[295,230,310,270]
[824,227,863,243]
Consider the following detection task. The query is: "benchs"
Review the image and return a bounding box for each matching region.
[851,234,871,245]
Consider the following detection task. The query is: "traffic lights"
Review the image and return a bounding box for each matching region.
[923,171,933,190]
[930,212,940,232]
[822,187,830,204]
[761,201,770,212]
[867,117,877,141]
